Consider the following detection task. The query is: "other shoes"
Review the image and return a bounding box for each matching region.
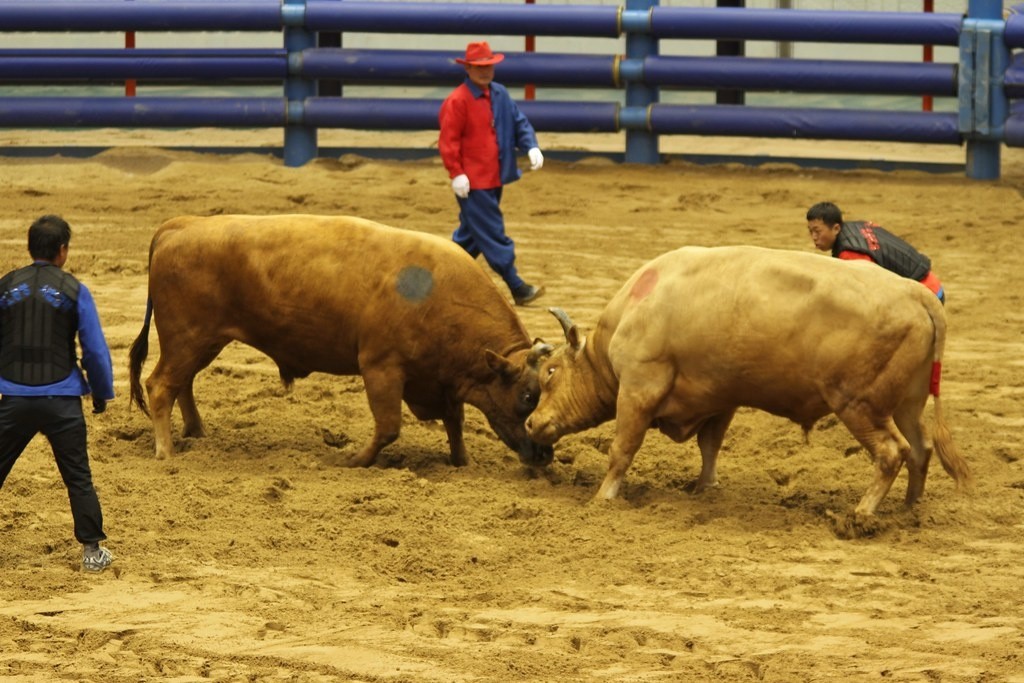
[512,284,544,305]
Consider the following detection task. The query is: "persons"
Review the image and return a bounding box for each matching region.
[807,201,946,306]
[438,42,548,306]
[0,214,117,575]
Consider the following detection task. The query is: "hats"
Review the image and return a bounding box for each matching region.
[456,43,504,66]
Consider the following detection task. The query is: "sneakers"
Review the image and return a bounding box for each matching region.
[82,547,112,573]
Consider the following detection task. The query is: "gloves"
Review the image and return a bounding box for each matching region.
[452,174,470,199]
[527,147,544,170]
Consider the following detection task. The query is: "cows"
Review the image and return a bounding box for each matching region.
[523,245,973,517]
[128,214,554,468]
